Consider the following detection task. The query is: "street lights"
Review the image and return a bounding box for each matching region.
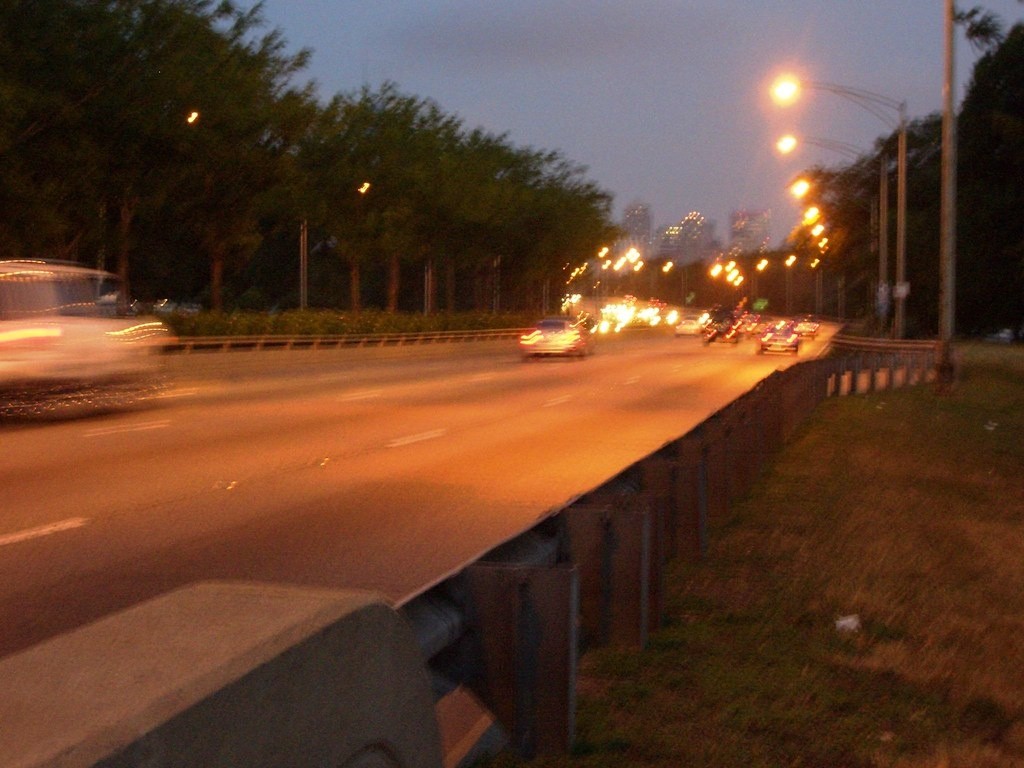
[788,179,878,329]
[770,74,907,343]
[773,135,890,337]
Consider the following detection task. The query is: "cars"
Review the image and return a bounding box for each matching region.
[0,259,171,419]
[520,320,593,358]
[676,311,819,354]
